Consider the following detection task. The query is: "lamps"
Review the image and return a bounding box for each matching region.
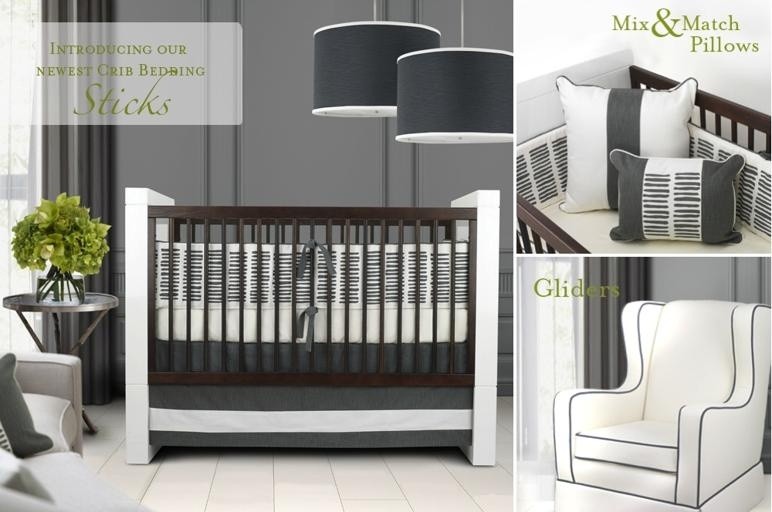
[311,1,441,119]
[395,1,513,147]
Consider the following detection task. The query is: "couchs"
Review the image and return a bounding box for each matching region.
[0,352,151,512]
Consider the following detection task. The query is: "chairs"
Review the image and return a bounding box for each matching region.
[551,301,770,512]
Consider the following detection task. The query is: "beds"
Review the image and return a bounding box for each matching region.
[517,121,770,253]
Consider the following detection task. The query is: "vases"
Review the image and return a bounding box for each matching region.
[32,265,85,305]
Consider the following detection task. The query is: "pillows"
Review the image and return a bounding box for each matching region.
[0,352,54,459]
[609,147,744,246]
[554,74,698,215]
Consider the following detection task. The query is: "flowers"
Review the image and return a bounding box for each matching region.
[10,192,112,301]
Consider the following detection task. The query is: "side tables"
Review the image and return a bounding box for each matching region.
[3,292,119,434]
